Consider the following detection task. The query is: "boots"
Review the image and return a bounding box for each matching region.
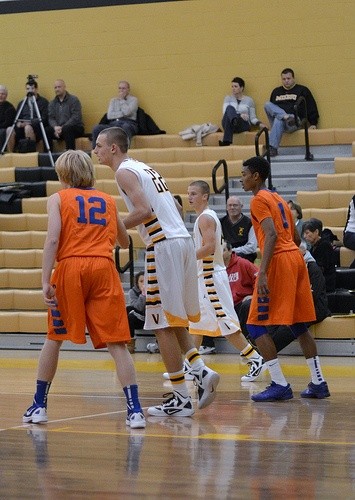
[127,338,136,354]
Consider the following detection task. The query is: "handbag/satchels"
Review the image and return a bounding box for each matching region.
[19,136,37,153]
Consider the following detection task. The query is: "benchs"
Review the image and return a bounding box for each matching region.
[0,127,355,358]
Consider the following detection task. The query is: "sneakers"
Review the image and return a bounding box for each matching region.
[190,366,220,409]
[287,115,299,125]
[199,345,215,355]
[240,355,267,382]
[146,343,159,353]
[270,147,278,157]
[300,380,331,399]
[126,406,146,428]
[163,365,194,380]
[23,403,49,422]
[251,381,294,402]
[146,390,194,417]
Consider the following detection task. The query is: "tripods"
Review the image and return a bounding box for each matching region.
[1,79,54,167]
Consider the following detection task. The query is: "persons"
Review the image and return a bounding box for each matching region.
[93,127,220,417]
[6,81,49,154]
[163,180,266,382]
[219,77,265,146]
[92,81,139,149]
[23,150,146,429]
[272,243,330,352]
[126,271,146,352]
[0,85,16,148]
[198,239,259,354]
[303,218,337,292]
[343,194,355,250]
[263,68,319,157]
[219,196,258,264]
[43,79,84,153]
[239,157,331,402]
[287,200,312,251]
